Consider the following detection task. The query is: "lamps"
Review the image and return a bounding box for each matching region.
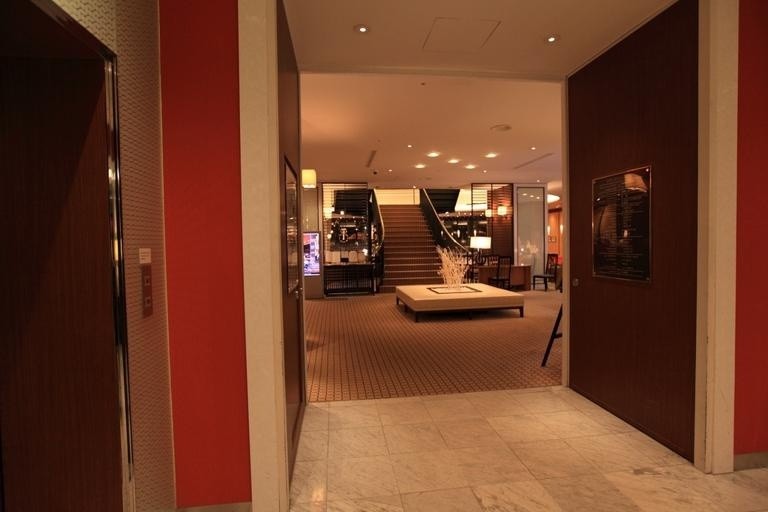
[497,206,507,216]
[470,236,492,265]
[302,169,317,188]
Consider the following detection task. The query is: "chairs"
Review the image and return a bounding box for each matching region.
[488,255,499,266]
[488,256,512,290]
[533,254,558,291]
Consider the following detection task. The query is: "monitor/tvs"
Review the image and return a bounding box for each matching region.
[303,231,321,276]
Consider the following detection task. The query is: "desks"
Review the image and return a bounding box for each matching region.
[479,265,531,291]
